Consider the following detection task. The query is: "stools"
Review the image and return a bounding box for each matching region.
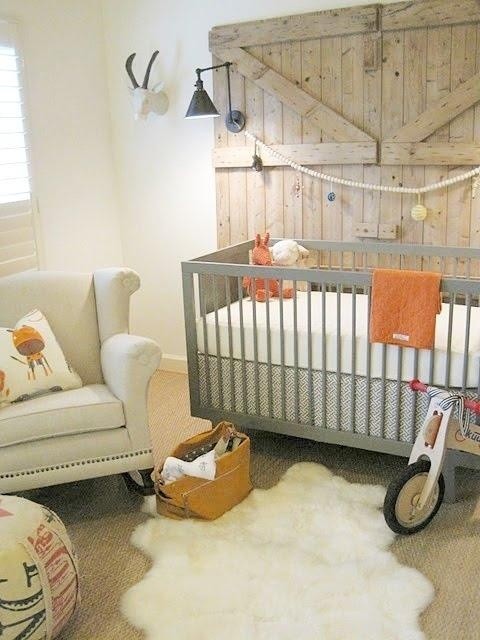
[2,494,86,640]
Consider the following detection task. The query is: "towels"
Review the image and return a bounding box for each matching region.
[367,267,443,354]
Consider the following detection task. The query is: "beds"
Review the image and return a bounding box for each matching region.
[181,235,480,505]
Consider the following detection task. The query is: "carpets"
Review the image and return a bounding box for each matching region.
[118,460,436,639]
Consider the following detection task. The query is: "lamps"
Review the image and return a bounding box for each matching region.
[186,59,246,136]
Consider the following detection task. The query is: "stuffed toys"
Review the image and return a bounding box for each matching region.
[269,240,310,293]
[242,233,294,302]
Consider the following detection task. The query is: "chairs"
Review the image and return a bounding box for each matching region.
[1,259,165,521]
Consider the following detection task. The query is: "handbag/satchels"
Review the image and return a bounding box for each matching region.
[150,421,254,522]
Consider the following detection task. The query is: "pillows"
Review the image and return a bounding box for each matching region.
[1,309,84,416]
